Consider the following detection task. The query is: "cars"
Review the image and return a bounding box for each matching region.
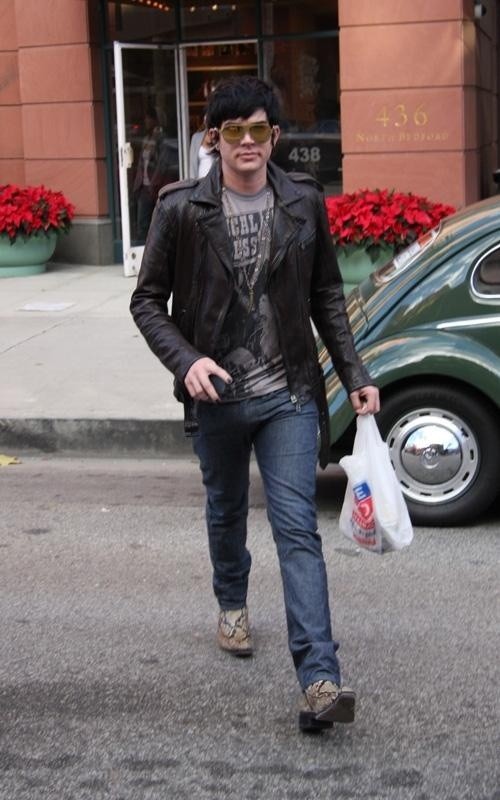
[315,192,500,527]
[277,119,342,179]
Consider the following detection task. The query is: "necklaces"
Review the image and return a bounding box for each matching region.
[221,184,271,316]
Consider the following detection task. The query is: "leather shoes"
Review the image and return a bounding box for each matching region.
[218,606,252,657]
[299,680,356,733]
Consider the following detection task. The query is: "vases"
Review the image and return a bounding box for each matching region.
[1,228,57,276]
[331,244,395,295]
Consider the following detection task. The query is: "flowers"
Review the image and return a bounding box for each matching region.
[324,189,456,256]
[0,179,78,243]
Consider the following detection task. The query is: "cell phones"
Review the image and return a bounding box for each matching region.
[209,370,228,396]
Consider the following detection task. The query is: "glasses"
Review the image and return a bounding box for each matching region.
[221,123,273,144]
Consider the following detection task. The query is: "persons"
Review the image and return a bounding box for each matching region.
[129,76,382,734]
[189,109,218,180]
[132,106,169,231]
